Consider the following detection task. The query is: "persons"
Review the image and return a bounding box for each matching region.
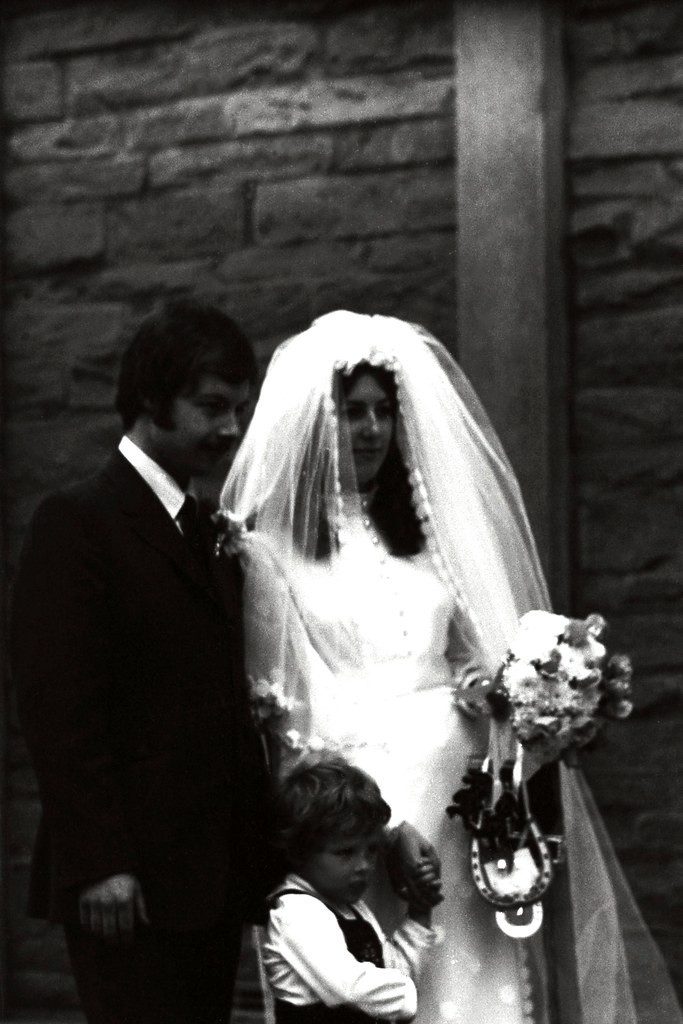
[9,300,314,1024]
[252,757,442,1024]
[216,309,683,1024]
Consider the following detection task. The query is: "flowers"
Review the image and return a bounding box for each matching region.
[244,676,291,729]
[451,609,639,768]
[213,509,250,557]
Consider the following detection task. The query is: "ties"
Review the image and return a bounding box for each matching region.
[175,494,200,537]
